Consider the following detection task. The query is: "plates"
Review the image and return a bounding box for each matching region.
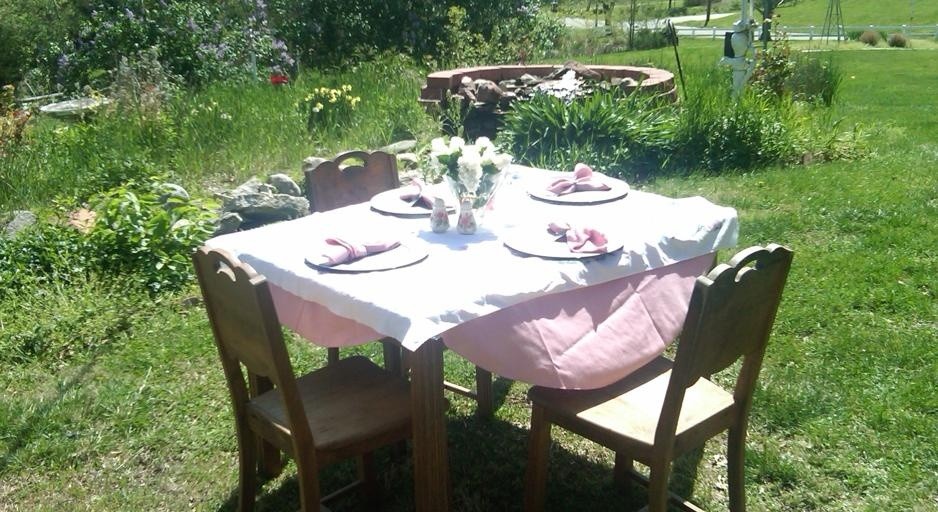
[525,175,630,204]
[370,185,456,215]
[305,228,430,273]
[503,226,623,259]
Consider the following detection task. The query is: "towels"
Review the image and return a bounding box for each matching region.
[548,163,611,198]
[524,242,794,512]
[400,179,439,210]
[547,221,609,254]
[309,234,400,267]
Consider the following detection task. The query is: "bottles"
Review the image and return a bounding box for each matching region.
[431,195,476,234]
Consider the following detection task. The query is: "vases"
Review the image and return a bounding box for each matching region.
[459,197,477,235]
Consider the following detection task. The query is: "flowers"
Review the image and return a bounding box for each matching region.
[430,135,513,200]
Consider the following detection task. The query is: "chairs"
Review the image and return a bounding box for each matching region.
[304,150,400,377]
[190,245,451,512]
